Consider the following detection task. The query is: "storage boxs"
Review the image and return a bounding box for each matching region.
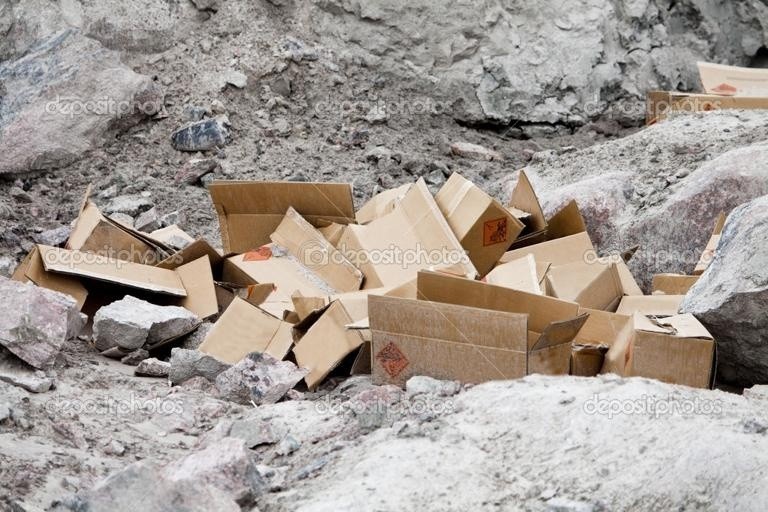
[10,170,727,392]
[646,62,767,125]
